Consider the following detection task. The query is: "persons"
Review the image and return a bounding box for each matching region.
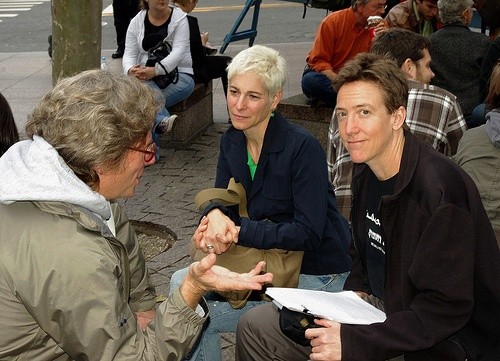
[169,40,357,361]
[171,0,233,124]
[0,69,274,361]
[384,0,440,38]
[122,0,195,163]
[450,58,500,249]
[468,34,500,128]
[234,51,500,361]
[369,27,469,158]
[425,0,500,127]
[301,0,390,100]
[112,0,142,58]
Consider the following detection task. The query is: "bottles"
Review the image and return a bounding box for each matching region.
[101,57,109,71]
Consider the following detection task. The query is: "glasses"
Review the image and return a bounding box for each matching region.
[365,4,387,11]
[127,142,156,163]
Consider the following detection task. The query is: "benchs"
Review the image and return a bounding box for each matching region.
[276,93,334,149]
[160,80,214,148]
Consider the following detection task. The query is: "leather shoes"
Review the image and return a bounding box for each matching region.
[112,47,124,58]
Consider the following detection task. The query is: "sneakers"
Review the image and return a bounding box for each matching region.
[155,114,178,138]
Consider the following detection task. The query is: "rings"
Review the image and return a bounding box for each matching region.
[207,244,214,249]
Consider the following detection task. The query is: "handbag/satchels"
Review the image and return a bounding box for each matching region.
[193,177,304,308]
[145,40,179,90]
